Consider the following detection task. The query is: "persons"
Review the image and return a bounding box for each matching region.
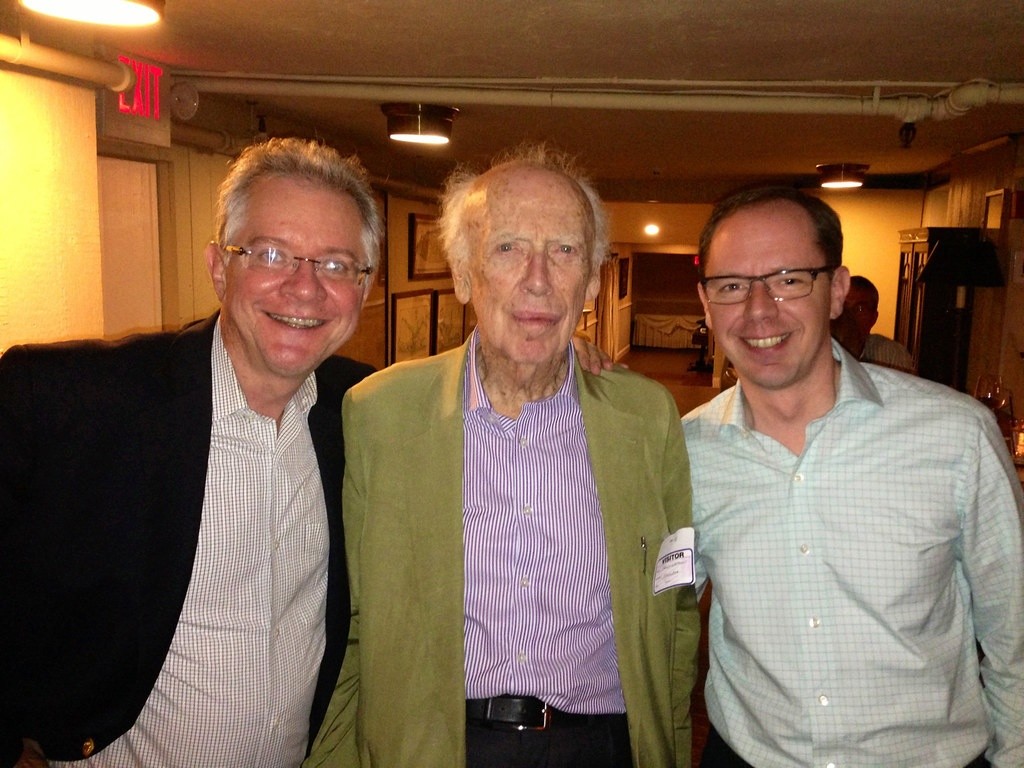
[831,276,912,373]
[0,135,630,768]
[681,186,1024,768]
[300,149,700,768]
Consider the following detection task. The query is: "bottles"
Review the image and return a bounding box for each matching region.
[943,285,971,393]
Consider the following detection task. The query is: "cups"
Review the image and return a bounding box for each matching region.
[974,374,1006,415]
[1009,419,1024,483]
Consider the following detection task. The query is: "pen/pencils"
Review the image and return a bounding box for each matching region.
[639,536,647,574]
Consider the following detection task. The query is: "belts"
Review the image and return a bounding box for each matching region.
[466,693,599,732]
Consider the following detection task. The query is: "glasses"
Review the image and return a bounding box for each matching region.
[698,264,839,307]
[225,239,372,288]
